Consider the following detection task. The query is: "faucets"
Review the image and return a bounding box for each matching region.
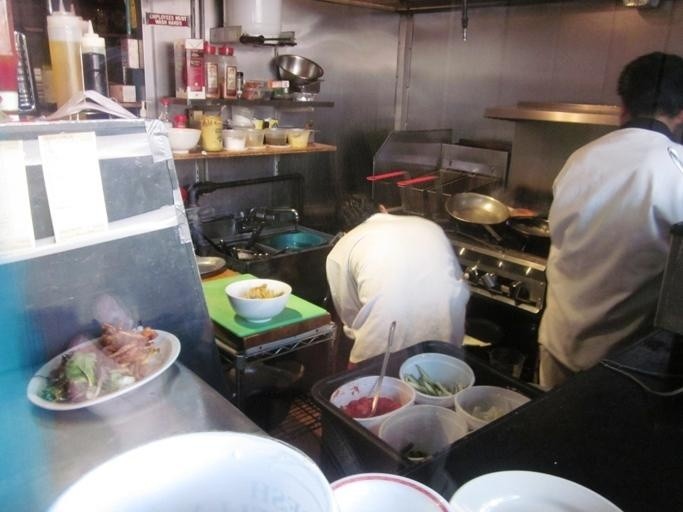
[264,208,300,232]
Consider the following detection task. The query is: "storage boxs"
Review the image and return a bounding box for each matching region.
[311,341,548,481]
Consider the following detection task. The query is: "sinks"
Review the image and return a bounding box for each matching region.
[263,232,332,252]
[217,242,276,263]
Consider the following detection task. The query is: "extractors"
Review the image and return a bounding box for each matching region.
[484,102,622,127]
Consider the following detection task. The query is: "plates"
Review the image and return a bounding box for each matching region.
[196,256,226,276]
[330,473,457,512]
[49,430,336,512]
[450,471,623,512]
[27,330,182,411]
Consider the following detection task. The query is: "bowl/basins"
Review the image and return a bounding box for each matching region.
[274,55,324,85]
[224,279,293,323]
[168,128,203,154]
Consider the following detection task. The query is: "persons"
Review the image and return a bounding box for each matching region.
[325,192,469,369]
[538,52,683,391]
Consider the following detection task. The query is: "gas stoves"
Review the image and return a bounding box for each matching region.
[447,237,546,315]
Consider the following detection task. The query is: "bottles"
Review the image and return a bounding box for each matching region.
[203,43,222,100]
[153,97,174,122]
[172,113,188,131]
[222,46,240,98]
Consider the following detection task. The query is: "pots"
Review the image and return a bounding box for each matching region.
[444,190,513,244]
[506,215,552,241]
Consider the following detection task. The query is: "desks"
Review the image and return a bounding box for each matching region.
[200,267,335,411]
